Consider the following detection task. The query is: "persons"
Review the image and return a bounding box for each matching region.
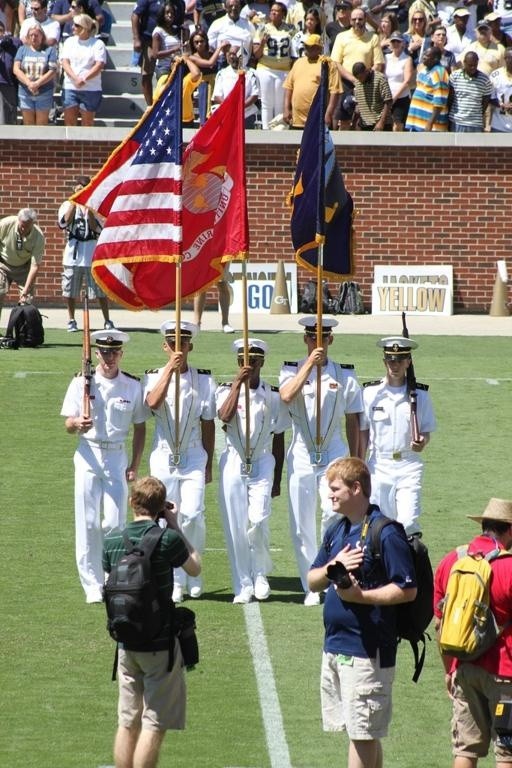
[359,337,437,552]
[434,498,512,767]
[0,207,46,305]
[278,316,365,606]
[214,338,292,604]
[142,319,218,602]
[101,475,201,768]
[59,330,154,603]
[307,457,419,767]
[193,279,235,334]
[55,177,119,331]
[1,0,512,133]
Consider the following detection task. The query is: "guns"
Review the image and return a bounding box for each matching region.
[402,311,428,444]
[82,293,95,419]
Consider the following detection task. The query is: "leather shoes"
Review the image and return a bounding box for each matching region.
[304,590,320,606]
[233,591,252,603]
[255,575,270,600]
[187,578,202,597]
[172,593,184,603]
[87,592,102,603]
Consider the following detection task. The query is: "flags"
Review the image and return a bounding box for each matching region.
[68,58,182,310]
[286,58,356,281]
[130,71,249,310]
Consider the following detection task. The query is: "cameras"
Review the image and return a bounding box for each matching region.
[324,560,365,604]
[159,499,174,519]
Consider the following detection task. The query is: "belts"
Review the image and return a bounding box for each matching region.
[299,435,338,441]
[378,451,410,459]
[158,441,201,448]
[229,447,268,454]
[82,439,123,449]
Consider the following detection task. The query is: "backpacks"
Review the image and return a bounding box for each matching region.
[6,301,48,347]
[333,281,365,314]
[102,526,167,643]
[300,280,333,314]
[371,518,434,640]
[438,545,511,661]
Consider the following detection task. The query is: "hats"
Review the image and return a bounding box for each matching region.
[376,337,418,362]
[301,33,324,46]
[161,321,199,343]
[233,338,266,358]
[484,13,502,23]
[453,9,471,16]
[390,34,403,42]
[466,498,511,523]
[91,329,129,352]
[299,317,338,340]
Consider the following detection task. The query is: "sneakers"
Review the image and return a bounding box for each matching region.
[104,321,116,330]
[224,325,235,333]
[68,320,77,332]
